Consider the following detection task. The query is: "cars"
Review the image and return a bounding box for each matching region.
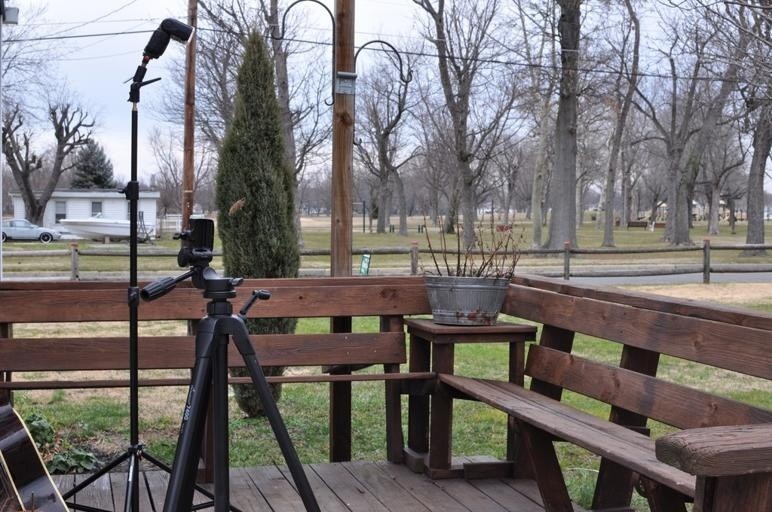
[2,218,63,244]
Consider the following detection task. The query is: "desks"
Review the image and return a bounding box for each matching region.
[401,316,538,469]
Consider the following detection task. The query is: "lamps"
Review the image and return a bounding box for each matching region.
[61,18,246,512]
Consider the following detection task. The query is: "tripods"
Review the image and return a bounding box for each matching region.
[162,301,322,512]
[60,105,241,512]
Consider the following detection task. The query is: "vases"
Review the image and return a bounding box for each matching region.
[421,274,513,327]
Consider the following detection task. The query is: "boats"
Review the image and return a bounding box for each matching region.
[57,211,157,243]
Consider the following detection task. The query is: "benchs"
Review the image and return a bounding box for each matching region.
[627,222,647,231]
[649,221,665,232]
[0,273,437,464]
[439,266,772,512]
